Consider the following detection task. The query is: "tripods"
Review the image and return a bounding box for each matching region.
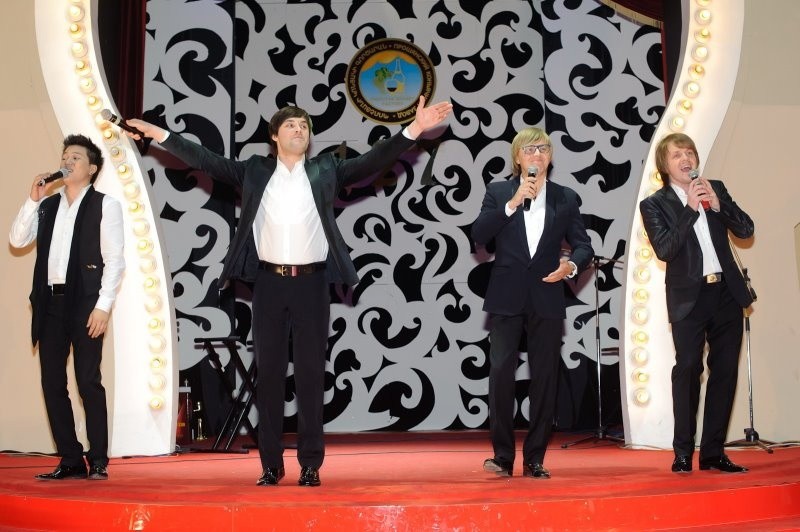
[722,269,791,453]
[560,258,625,450]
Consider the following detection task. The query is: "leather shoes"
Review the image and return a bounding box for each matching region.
[257,464,285,486]
[483,455,513,476]
[699,453,749,472]
[88,463,108,480]
[34,458,88,480]
[298,464,321,486]
[671,455,693,472]
[523,461,551,478]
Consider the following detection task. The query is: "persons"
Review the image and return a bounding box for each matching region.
[9,135,126,481]
[641,133,755,473]
[472,127,595,478]
[122,96,453,486]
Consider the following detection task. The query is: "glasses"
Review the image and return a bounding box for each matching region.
[521,143,551,155]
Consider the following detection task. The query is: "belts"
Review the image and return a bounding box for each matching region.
[703,273,722,284]
[259,262,313,277]
[47,284,65,296]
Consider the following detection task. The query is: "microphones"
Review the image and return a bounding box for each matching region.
[37,167,69,188]
[687,169,712,213]
[522,165,539,211]
[99,108,146,139]
